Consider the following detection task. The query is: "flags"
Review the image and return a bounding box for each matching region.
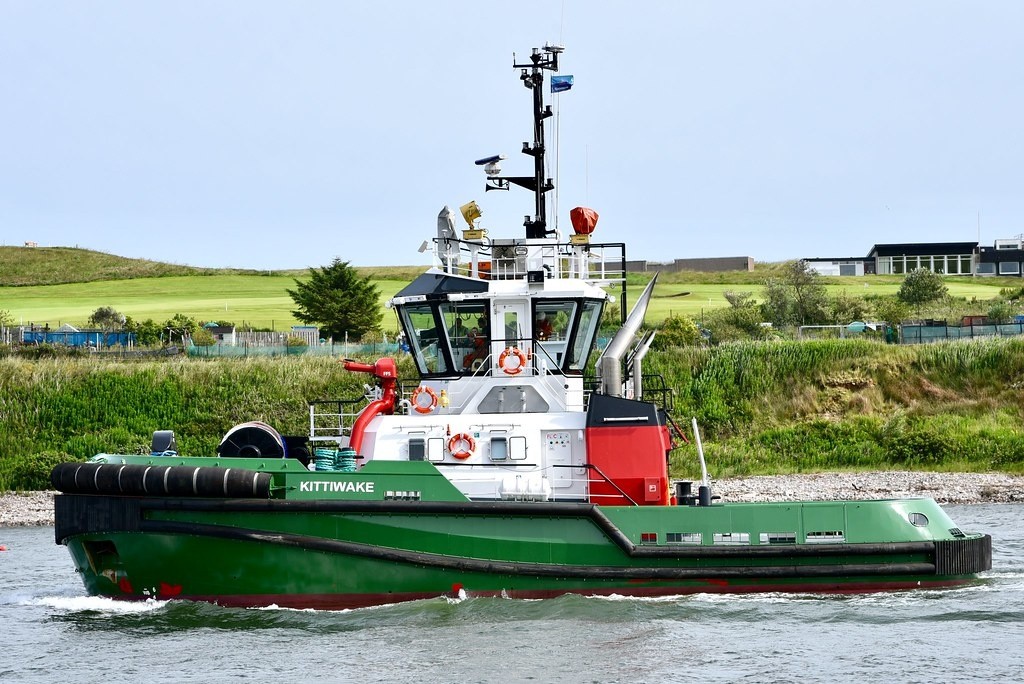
[551,75,574,93]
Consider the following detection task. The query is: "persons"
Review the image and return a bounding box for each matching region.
[533,215,558,238]
[462,327,488,373]
[449,318,471,345]
[478,318,488,346]
[523,215,536,239]
[536,311,552,341]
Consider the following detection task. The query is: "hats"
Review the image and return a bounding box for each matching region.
[536,312,546,320]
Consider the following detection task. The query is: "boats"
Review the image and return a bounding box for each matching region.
[51,40,996,611]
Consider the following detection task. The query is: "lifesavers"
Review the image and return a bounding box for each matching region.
[499,347,526,375]
[411,387,436,412]
[448,434,476,457]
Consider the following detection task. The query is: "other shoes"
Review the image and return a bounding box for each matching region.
[458,368,467,372]
